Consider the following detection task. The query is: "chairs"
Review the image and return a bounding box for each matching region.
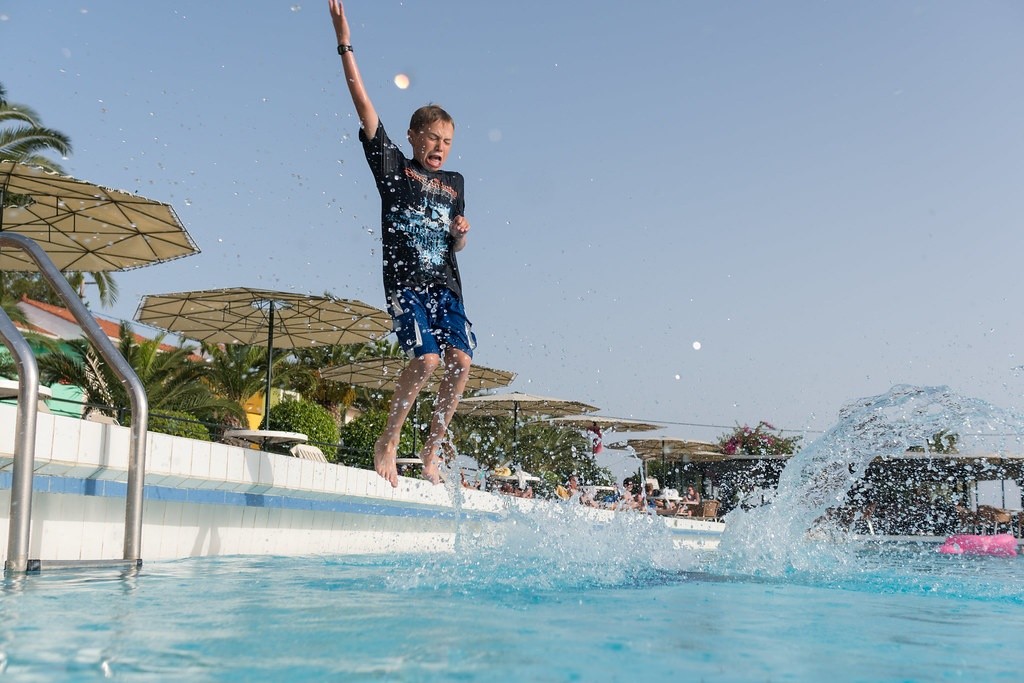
[696,500,720,522]
[973,504,1014,539]
[288,444,330,463]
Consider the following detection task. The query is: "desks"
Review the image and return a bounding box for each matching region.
[651,496,683,514]
[224,429,309,452]
[396,457,426,478]
[575,486,615,504]
[488,475,541,491]
[0,379,52,404]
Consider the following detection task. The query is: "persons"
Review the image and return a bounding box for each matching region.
[328,1,481,492]
[438,440,1024,544]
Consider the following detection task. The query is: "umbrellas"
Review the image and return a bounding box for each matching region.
[0,155,200,282]
[134,287,396,450]
[534,417,1024,511]
[447,390,600,498]
[316,350,516,482]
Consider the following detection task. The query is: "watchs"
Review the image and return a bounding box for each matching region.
[337,44,353,55]
